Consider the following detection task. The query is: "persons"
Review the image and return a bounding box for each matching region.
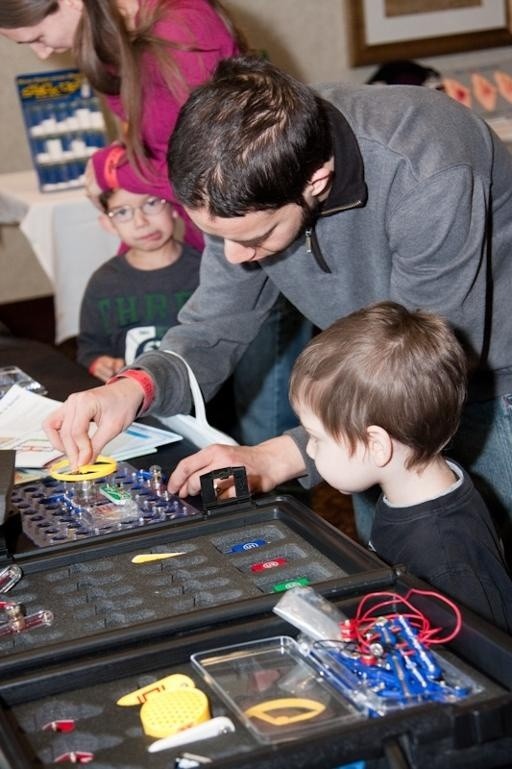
[290,302,512,636]
[77,185,239,435]
[43,54,512,550]
[0,0,317,509]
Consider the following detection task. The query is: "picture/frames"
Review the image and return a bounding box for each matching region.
[343,0,512,70]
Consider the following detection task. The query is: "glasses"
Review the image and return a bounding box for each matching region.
[104,197,167,223]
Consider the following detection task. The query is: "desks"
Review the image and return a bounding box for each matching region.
[1,170,118,347]
